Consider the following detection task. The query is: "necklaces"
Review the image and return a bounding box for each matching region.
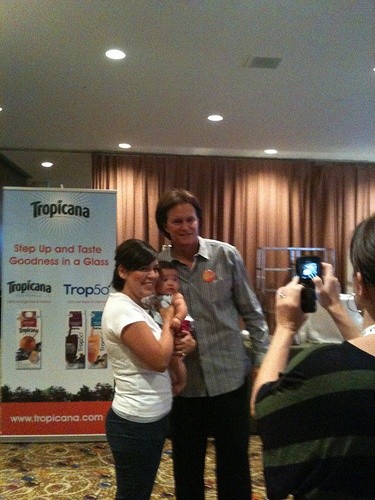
[362,324,375,337]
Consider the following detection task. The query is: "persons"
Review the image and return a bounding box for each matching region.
[141,261,195,397]
[101,239,197,500]
[250,214,375,500]
[155,191,269,500]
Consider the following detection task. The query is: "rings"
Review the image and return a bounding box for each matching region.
[279,291,286,298]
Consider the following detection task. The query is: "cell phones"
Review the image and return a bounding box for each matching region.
[297,256,321,313]
